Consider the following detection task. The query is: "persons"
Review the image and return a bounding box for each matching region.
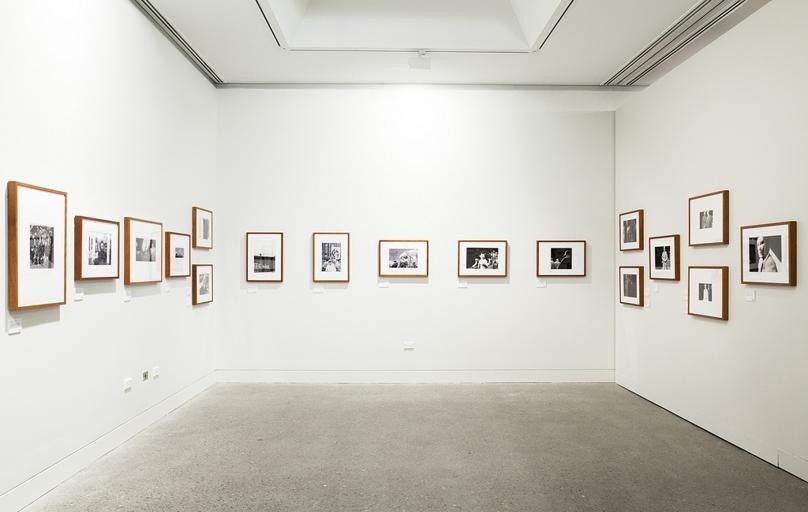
[93,239,108,265]
[392,250,571,269]
[323,244,341,271]
[621,211,782,300]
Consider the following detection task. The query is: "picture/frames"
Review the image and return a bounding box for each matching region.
[378,238,428,277]
[312,230,350,283]
[3,177,216,315]
[456,238,507,279]
[245,231,283,284]
[535,238,586,278]
[616,189,801,323]
